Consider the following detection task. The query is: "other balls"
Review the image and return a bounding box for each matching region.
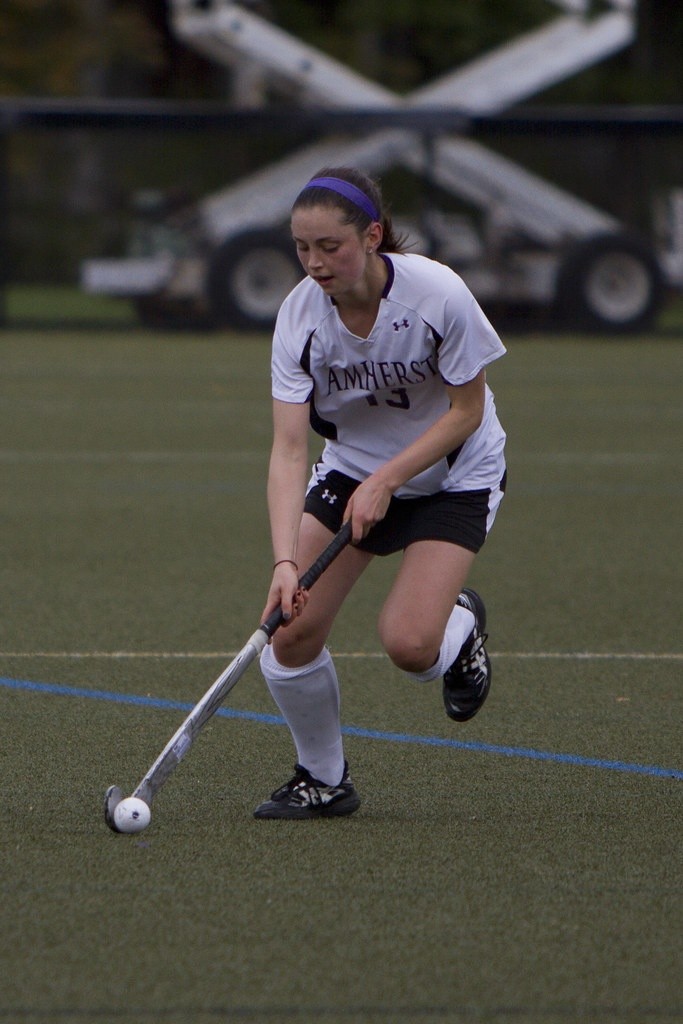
[114,797,152,831]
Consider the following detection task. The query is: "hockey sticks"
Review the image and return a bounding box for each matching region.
[103,515,353,831]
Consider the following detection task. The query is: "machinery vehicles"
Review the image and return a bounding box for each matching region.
[117,0,671,333]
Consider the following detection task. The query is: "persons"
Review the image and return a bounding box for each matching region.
[257,164,507,818]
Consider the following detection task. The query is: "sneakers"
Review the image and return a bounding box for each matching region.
[440,587,490,722]
[254,761,364,822]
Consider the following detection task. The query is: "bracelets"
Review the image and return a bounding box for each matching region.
[273,560,299,571]
